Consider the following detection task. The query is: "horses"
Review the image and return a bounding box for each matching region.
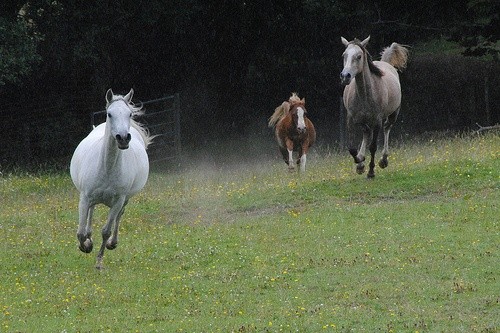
[268,93,316,173]
[340,36,408,179]
[69,89,160,271]
